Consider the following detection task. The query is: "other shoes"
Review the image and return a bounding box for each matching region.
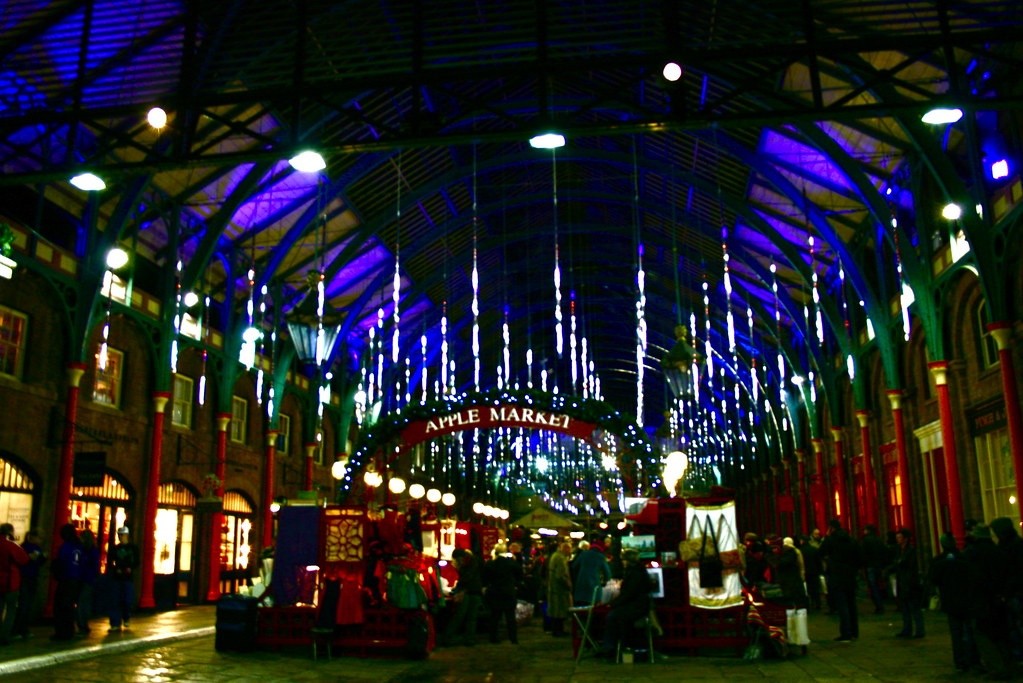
[10,633,23,641]
[26,632,34,638]
[108,626,122,632]
[971,662,987,672]
[833,633,858,641]
[552,629,569,638]
[124,622,128,627]
[49,635,71,641]
[896,632,911,637]
[0,639,9,646]
[914,634,925,639]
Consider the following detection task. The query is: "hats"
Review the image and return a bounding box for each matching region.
[968,523,992,540]
[118,527,129,534]
[940,531,956,548]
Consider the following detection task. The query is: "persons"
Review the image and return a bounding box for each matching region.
[0,521,100,644]
[106,526,137,632]
[603,548,651,659]
[927,517,1023,683]
[743,516,927,641]
[449,535,612,658]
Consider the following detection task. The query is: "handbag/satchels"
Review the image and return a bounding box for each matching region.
[678,514,743,588]
